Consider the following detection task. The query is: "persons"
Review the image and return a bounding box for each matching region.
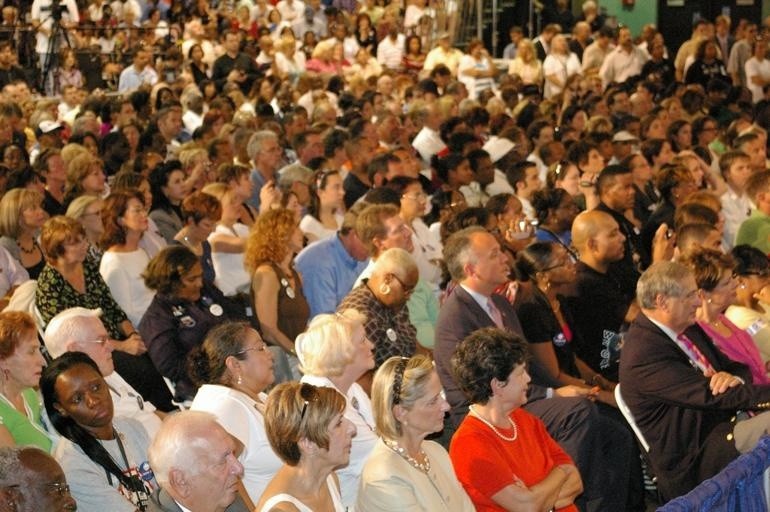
[727,21,759,101]
[448,325,585,512]
[1,445,78,512]
[543,34,582,101]
[36,216,179,413]
[247,131,294,208]
[33,82,81,152]
[212,30,265,95]
[604,72,702,105]
[621,259,770,499]
[366,187,401,210]
[8,165,46,203]
[504,26,525,58]
[675,20,723,84]
[30,120,66,165]
[216,163,259,228]
[513,242,617,408]
[444,208,587,388]
[736,168,770,258]
[746,38,770,103]
[714,16,737,73]
[557,211,676,385]
[722,244,770,368]
[719,152,757,248]
[243,208,310,380]
[254,382,357,512]
[640,32,671,75]
[62,153,105,215]
[734,135,766,170]
[336,75,455,169]
[30,1,79,81]
[467,149,495,206]
[645,167,698,267]
[597,26,648,93]
[684,191,726,235]
[675,203,718,231]
[533,0,576,35]
[544,160,599,209]
[392,147,421,179]
[350,153,403,215]
[299,168,348,245]
[401,35,428,73]
[508,39,543,102]
[593,165,641,296]
[168,190,253,325]
[81,1,215,170]
[361,0,459,43]
[97,188,157,330]
[0,0,31,142]
[34,148,73,217]
[674,150,728,198]
[566,22,595,64]
[43,307,166,442]
[358,356,475,511]
[201,179,283,328]
[435,226,600,467]
[620,153,661,229]
[457,38,498,100]
[352,13,378,56]
[189,321,284,512]
[110,172,167,262]
[0,142,31,194]
[0,246,43,328]
[350,50,377,77]
[353,201,441,361]
[217,0,337,31]
[424,32,464,81]
[294,202,374,316]
[39,351,155,510]
[581,26,617,76]
[639,22,669,59]
[756,25,770,60]
[431,154,472,208]
[267,32,342,92]
[684,40,734,99]
[506,161,542,219]
[670,245,770,385]
[529,185,579,264]
[66,196,106,269]
[486,193,535,248]
[377,18,409,75]
[386,175,446,294]
[581,94,770,154]
[532,24,562,63]
[1,310,55,455]
[679,223,727,260]
[455,103,568,162]
[148,409,250,512]
[294,309,378,512]
[282,191,302,220]
[0,188,48,280]
[752,82,770,162]
[570,139,604,175]
[643,139,674,175]
[147,159,186,247]
[577,1,605,32]
[214,94,336,164]
[331,247,436,401]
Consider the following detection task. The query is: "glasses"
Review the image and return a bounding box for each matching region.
[738,269,767,279]
[24,485,72,499]
[298,383,316,421]
[233,341,268,356]
[535,254,572,273]
[81,208,108,217]
[401,193,428,202]
[701,126,719,134]
[386,272,415,293]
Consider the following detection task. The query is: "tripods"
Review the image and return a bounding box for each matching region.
[39,25,79,95]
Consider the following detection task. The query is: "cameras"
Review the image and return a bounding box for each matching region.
[40,0,69,23]
[518,218,540,234]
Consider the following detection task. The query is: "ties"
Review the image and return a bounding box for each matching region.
[678,333,712,373]
[487,298,505,332]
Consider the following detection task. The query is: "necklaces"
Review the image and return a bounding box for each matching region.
[467,405,519,441]
[386,439,431,473]
[16,237,37,255]
[283,272,296,282]
[706,319,723,328]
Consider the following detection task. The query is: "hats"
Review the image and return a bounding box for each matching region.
[34,120,65,137]
[612,129,639,145]
